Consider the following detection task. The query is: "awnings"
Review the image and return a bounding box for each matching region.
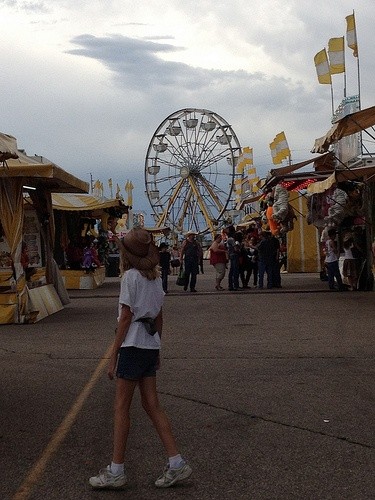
[311,106,375,173]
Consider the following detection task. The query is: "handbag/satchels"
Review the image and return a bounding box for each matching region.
[170,259,180,267]
[176,270,185,286]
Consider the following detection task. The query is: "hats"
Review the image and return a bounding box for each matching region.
[115,226,159,270]
[158,243,169,248]
[186,230,198,234]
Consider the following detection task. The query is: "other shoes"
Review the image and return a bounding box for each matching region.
[228,287,245,291]
[215,285,225,291]
[184,286,188,291]
[259,286,263,288]
[254,285,257,288]
[190,289,197,292]
[243,285,251,288]
[163,291,168,296]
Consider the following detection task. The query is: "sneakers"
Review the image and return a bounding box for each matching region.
[154,460,193,488]
[89,465,128,488]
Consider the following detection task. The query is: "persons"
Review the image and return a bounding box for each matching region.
[68,231,285,295]
[325,228,348,292]
[88,227,192,488]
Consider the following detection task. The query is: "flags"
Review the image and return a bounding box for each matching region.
[235,132,293,204]
[314,14,358,84]
[93,178,134,208]
